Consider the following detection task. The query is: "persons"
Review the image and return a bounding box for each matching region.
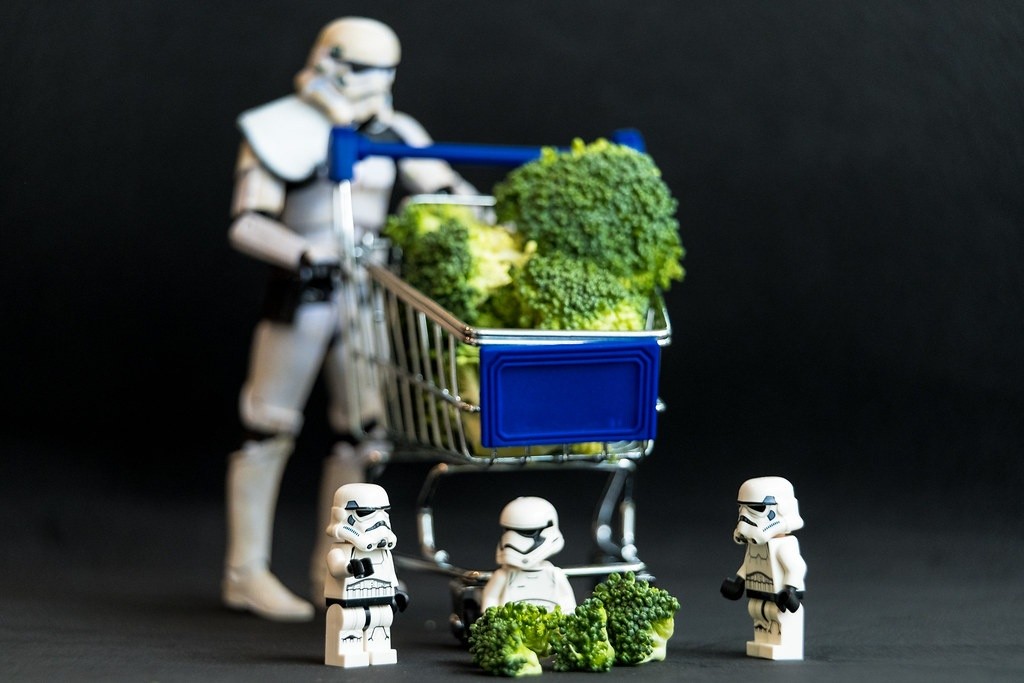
[479,497,578,618]
[319,483,400,668]
[718,477,806,661]
[220,19,493,618]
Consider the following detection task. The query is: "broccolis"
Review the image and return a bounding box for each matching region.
[468,571,682,676]
[376,135,685,455]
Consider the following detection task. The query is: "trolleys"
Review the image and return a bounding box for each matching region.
[327,125,677,655]
